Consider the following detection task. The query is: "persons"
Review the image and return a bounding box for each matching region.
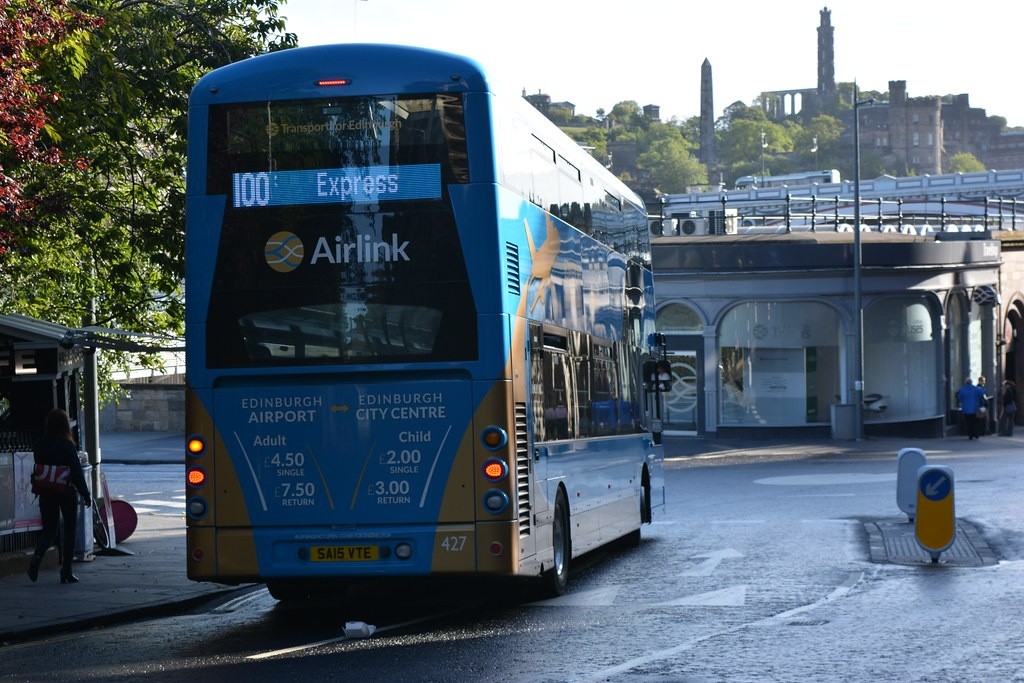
[28,409,91,583]
[958,378,981,440]
[998,380,1016,436]
[977,376,994,409]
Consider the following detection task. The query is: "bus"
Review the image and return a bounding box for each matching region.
[186,42,674,608]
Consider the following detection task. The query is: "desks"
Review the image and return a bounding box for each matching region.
[863,397,878,407]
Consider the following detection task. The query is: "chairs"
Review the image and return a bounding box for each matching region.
[835,393,893,436]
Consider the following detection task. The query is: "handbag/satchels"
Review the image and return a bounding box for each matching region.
[975,406,987,418]
[1005,402,1017,413]
[31,463,72,497]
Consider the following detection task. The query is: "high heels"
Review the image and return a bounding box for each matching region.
[61,565,78,583]
[28,555,41,581]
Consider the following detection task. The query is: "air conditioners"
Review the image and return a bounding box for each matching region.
[646,209,738,236]
